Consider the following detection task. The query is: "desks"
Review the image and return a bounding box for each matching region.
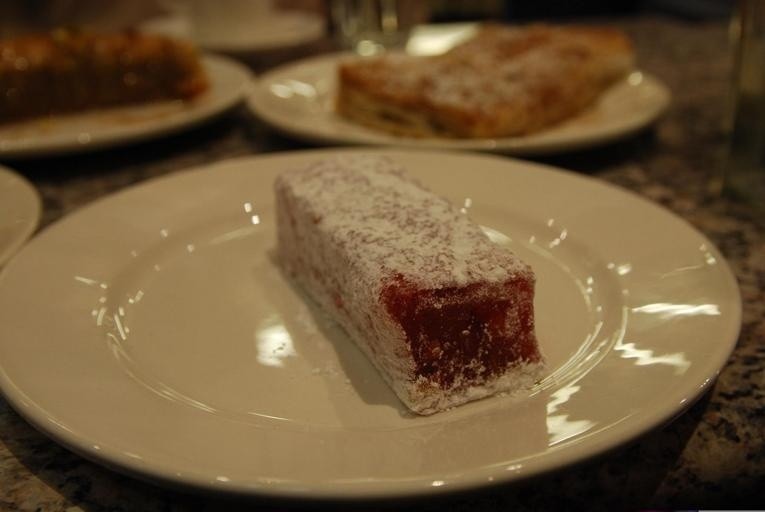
[0,14,765,510]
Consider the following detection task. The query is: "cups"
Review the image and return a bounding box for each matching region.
[328,1,411,56]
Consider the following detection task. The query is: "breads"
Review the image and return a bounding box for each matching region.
[334,21,638,138]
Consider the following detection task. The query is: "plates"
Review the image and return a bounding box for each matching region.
[142,9,324,55]
[0,167,45,268]
[245,49,672,159]
[2,55,255,159]
[2,151,747,499]
